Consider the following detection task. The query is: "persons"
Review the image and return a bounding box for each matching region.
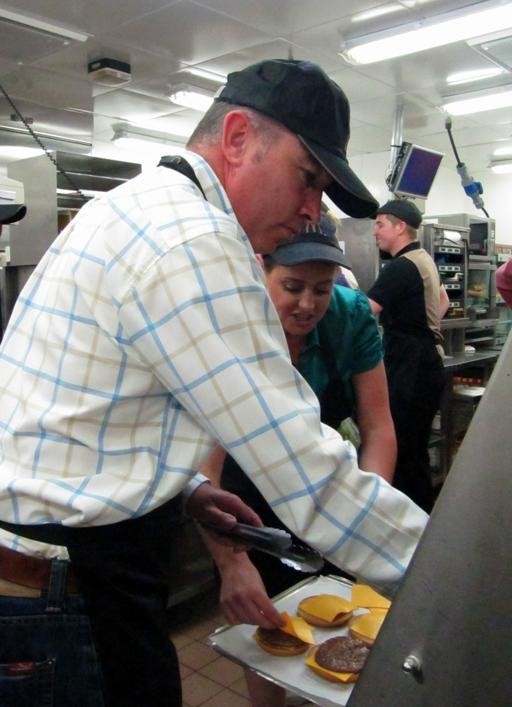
[363,197,453,516]
[0,58,432,706]
[190,201,400,707]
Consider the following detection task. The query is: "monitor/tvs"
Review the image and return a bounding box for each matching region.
[388,138,445,201]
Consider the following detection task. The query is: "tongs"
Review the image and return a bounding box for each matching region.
[196,518,325,573]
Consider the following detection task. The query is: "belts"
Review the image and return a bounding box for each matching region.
[0,547,79,593]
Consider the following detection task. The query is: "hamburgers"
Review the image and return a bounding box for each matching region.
[255,595,374,683]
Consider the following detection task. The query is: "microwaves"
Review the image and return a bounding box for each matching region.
[421,212,497,260]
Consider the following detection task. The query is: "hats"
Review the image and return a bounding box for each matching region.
[266,211,353,271]
[213,57,380,220]
[367,199,424,230]
